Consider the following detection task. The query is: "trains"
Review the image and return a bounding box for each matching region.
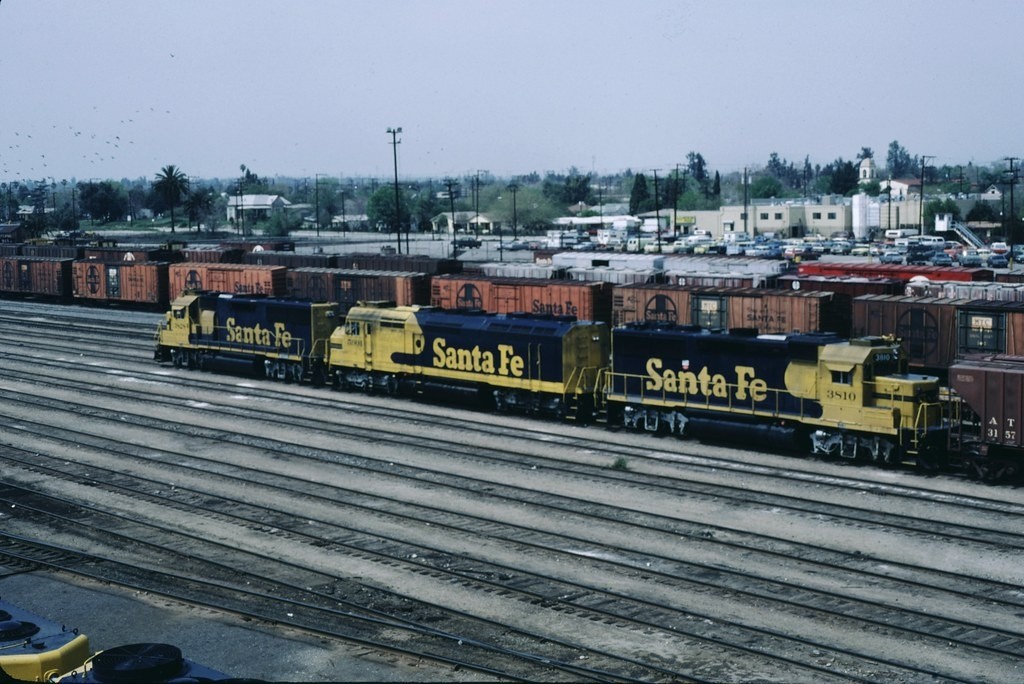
[151,278,969,474]
[0,219,1023,473]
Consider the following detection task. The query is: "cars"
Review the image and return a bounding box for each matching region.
[879,250,903,265]
[987,255,1008,269]
[956,250,983,267]
[450,237,483,249]
[932,253,953,266]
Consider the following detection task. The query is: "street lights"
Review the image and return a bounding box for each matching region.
[386,126,403,255]
